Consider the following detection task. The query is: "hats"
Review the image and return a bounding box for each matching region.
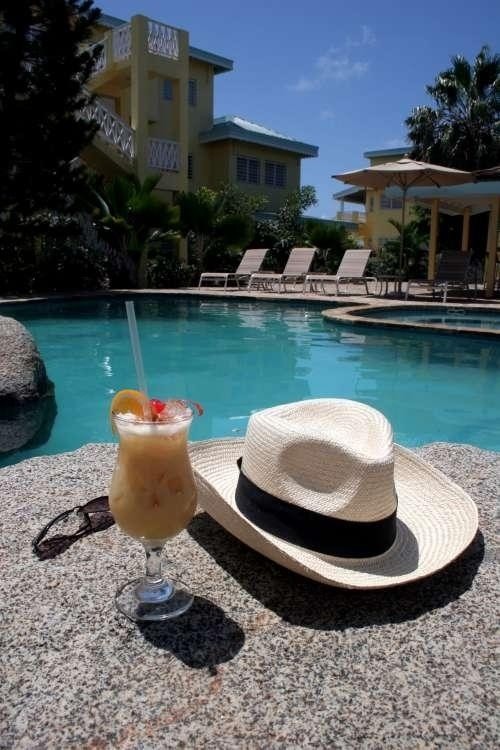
[185,399,478,589]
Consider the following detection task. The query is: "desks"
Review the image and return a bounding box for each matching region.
[378,275,403,298]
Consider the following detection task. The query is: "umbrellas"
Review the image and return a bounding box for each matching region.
[331,152,472,294]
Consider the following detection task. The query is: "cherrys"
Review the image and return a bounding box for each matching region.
[148,400,166,422]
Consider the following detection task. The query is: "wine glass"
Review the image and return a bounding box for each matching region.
[107,405,194,621]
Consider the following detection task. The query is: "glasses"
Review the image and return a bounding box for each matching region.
[31,497,109,554]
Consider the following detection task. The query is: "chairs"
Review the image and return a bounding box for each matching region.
[404,251,473,302]
[198,249,376,296]
